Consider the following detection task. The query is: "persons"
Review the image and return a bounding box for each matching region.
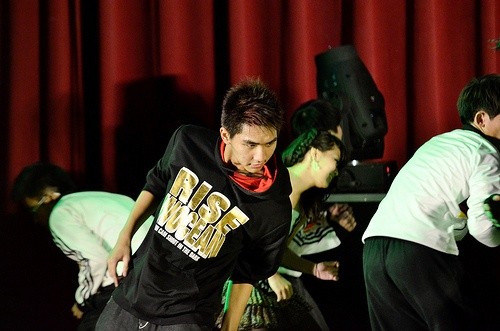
[288,99,370,330]
[96,74,291,331]
[12,160,154,331]
[360,71,500,331]
[216,127,346,330]
